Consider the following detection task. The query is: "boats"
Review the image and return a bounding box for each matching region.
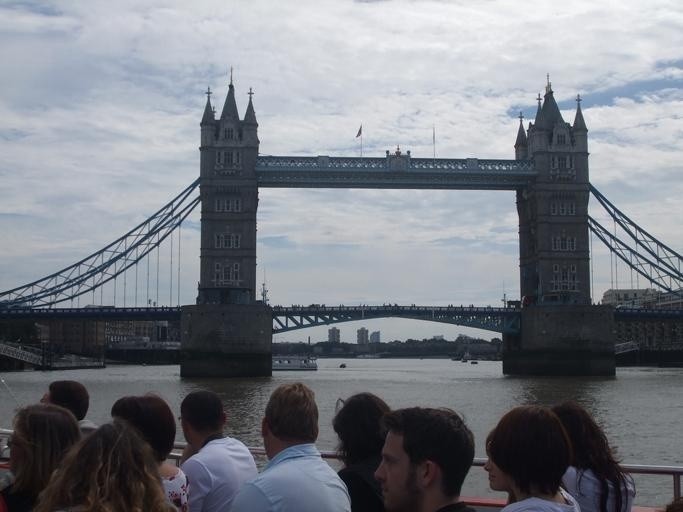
[451,351,471,360]
[109,336,181,364]
[271,353,319,370]
